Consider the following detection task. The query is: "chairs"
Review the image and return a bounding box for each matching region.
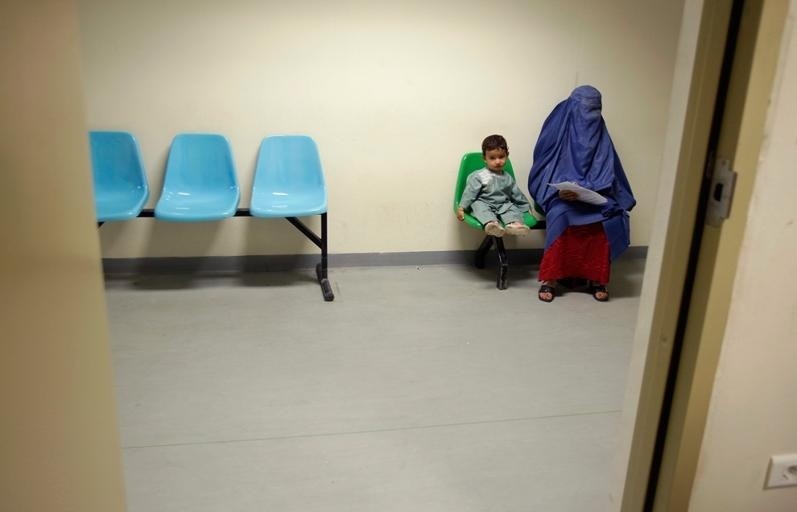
[455,152,536,289]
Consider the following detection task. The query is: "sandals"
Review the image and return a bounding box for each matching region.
[588,280,610,302]
[538,283,556,302]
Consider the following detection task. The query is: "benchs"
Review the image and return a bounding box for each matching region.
[84,128,334,302]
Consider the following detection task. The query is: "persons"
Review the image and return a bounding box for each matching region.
[528,82,636,302]
[457,133,531,239]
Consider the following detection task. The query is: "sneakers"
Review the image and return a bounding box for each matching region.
[505,222,530,237]
[483,221,505,238]
